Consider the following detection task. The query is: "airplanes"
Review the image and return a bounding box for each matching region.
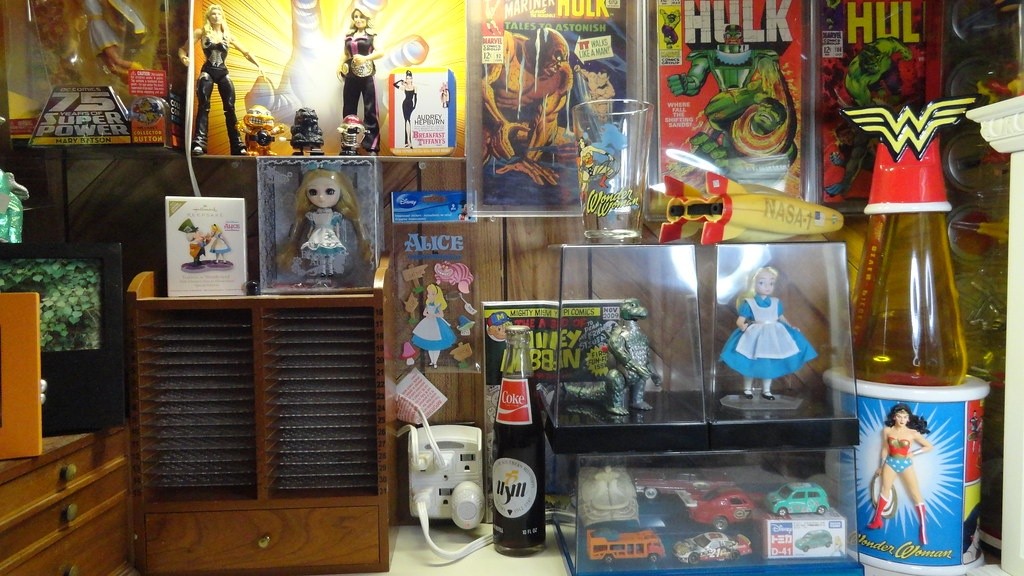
[651,171,845,245]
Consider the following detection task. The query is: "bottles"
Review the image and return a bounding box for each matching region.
[846,214,965,386]
[493,325,546,557]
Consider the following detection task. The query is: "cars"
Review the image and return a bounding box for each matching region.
[636,468,736,499]
[795,530,833,553]
[687,487,767,532]
[670,532,753,566]
[764,482,832,518]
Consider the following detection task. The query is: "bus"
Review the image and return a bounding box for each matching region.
[585,527,665,564]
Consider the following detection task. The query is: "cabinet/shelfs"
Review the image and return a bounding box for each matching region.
[0,424,134,576]
[124,257,389,574]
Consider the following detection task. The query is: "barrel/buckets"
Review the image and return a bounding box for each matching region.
[821,366,991,575]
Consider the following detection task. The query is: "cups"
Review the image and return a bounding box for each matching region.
[572,98,655,245]
[863,136,954,213]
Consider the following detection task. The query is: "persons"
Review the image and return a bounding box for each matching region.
[72,0,144,85]
[178,4,270,157]
[275,164,375,287]
[393,71,418,148]
[867,403,933,546]
[718,264,818,400]
[337,6,385,156]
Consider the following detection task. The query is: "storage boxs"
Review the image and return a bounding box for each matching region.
[748,505,848,561]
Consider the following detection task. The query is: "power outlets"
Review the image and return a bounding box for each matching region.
[407,423,485,520]
